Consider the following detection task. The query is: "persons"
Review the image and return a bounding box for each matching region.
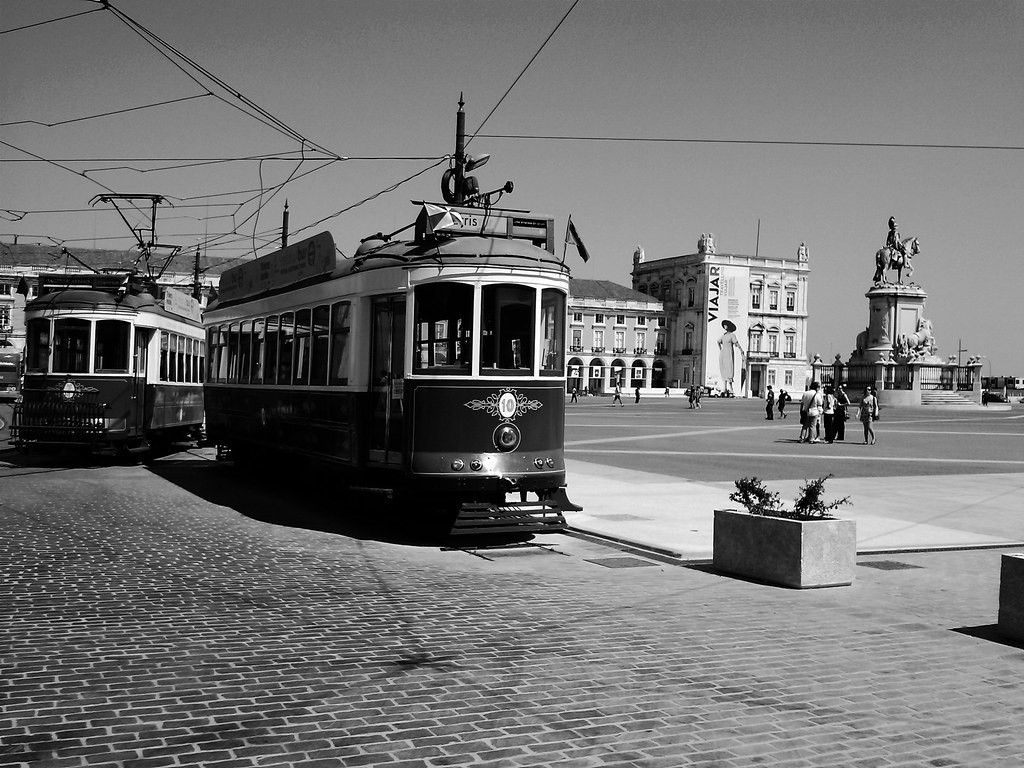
[765,385,774,420]
[666,386,669,397]
[613,382,624,407]
[717,319,744,391]
[798,382,850,444]
[886,216,909,268]
[855,386,878,446]
[982,389,988,406]
[570,387,577,403]
[635,386,640,404]
[776,389,788,419]
[688,385,701,409]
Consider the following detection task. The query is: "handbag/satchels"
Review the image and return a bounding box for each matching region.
[872,396,880,420]
[799,411,807,425]
[807,407,819,417]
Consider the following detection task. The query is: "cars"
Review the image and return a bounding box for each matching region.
[0,332,26,402]
[1018,398,1024,403]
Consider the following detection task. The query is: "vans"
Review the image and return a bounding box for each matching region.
[982,392,1006,402]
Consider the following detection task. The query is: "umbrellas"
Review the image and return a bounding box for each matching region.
[741,356,746,390]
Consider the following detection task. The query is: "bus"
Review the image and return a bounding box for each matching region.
[206,321,331,386]
[198,199,583,539]
[6,269,208,465]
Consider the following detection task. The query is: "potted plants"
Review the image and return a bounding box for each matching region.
[712,471,856,590]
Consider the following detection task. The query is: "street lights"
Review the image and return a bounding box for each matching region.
[983,356,992,391]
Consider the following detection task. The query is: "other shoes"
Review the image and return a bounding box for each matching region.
[863,442,868,444]
[798,439,804,443]
[824,440,832,444]
[814,438,821,443]
[784,414,787,419]
[766,417,773,420]
[810,440,812,444]
[871,435,876,445]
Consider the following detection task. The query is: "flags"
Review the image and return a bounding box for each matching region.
[424,203,464,231]
[567,219,590,262]
[16,276,29,300]
